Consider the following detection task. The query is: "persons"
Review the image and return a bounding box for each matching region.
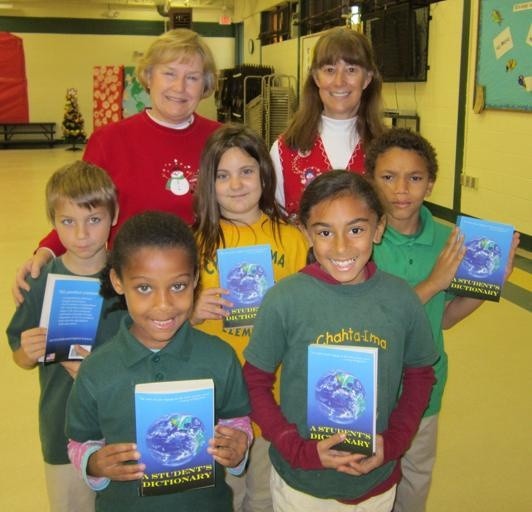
[8,26,228,309]
[266,29,392,265]
[239,168,442,512]
[6,159,120,512]
[62,206,256,512]
[174,121,318,512]
[350,125,521,511]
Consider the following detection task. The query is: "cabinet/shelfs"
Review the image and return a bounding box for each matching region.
[0,122,56,147]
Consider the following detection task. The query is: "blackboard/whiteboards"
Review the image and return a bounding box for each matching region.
[475,0,531,114]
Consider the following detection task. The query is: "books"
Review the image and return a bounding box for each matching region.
[216,245,276,329]
[305,342,378,459]
[134,377,219,499]
[444,214,517,305]
[37,271,103,362]
